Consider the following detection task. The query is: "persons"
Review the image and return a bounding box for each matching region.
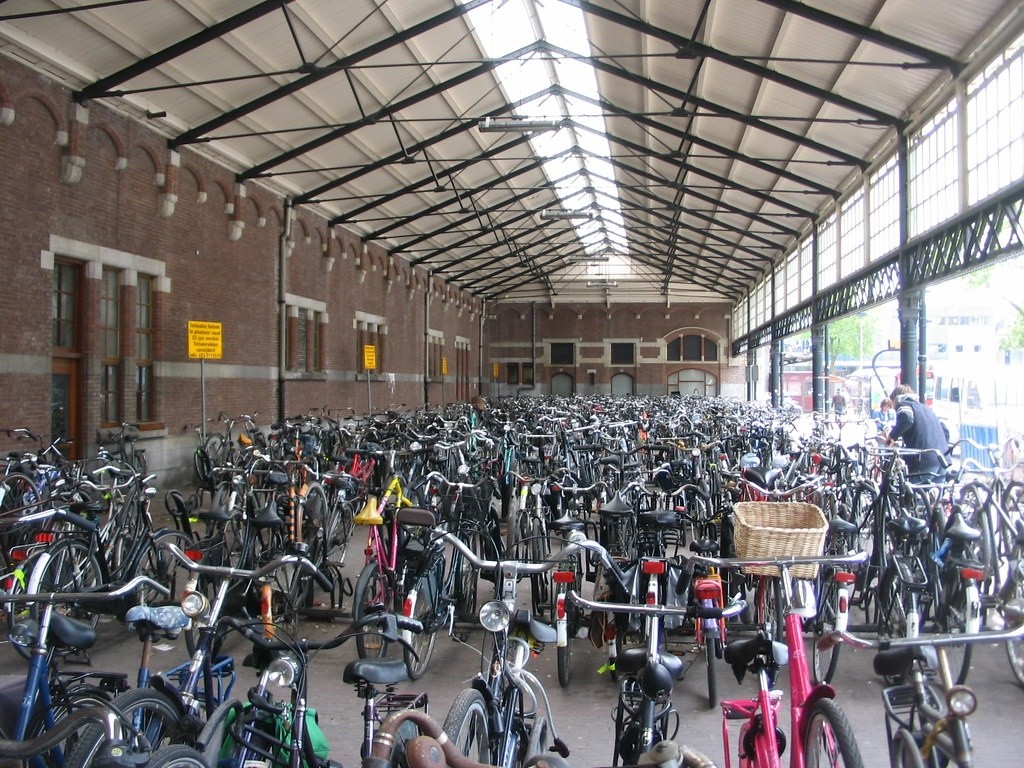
[874,384,952,505]
[831,388,850,423]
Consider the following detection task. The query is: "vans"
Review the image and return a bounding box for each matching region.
[933,368,1024,465]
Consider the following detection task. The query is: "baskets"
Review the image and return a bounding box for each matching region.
[439,478,493,523]
[733,502,827,581]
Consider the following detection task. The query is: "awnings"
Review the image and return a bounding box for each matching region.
[846,367,888,378]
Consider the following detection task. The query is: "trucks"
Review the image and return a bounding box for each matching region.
[867,369,934,419]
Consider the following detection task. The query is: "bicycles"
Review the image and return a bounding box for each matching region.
[1,391,1024,768]
